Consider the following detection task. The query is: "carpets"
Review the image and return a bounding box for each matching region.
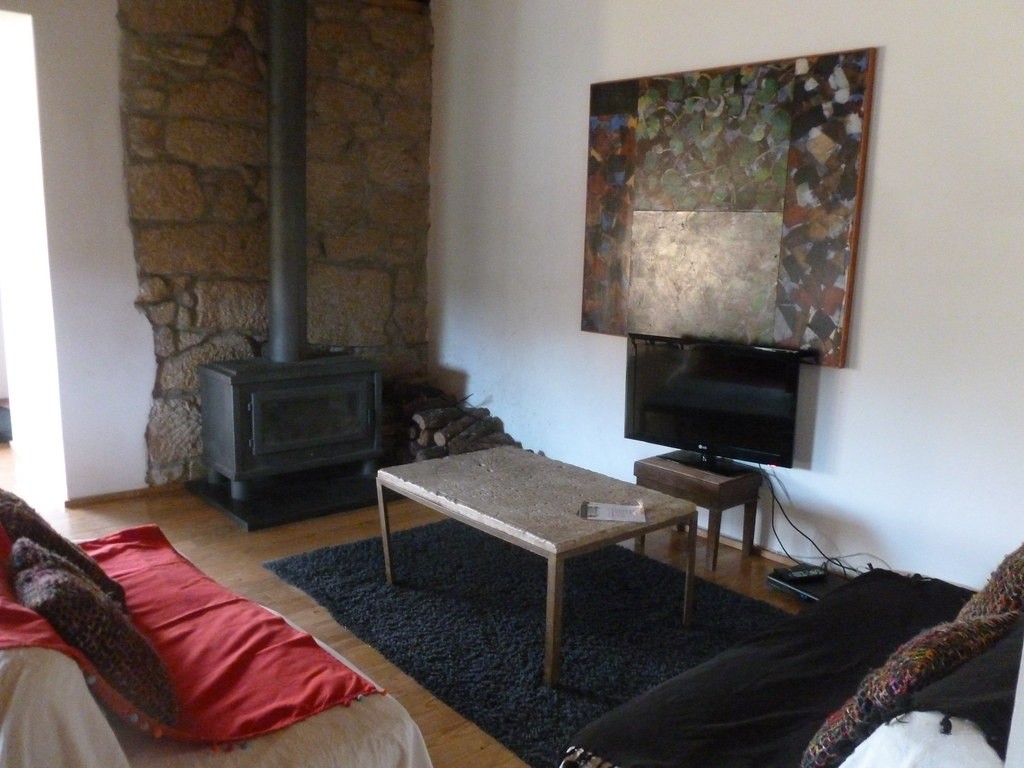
[260,519,795,768]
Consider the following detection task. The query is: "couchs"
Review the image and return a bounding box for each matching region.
[0,522,433,768]
[561,566,1024,768]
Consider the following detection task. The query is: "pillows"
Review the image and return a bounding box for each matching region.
[0,488,127,614]
[9,536,179,728]
[802,612,1024,768]
[953,545,1024,619]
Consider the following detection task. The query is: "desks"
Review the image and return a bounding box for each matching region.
[634,449,762,572]
[376,444,699,686]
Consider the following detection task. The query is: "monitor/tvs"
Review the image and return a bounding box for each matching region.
[625,333,799,477]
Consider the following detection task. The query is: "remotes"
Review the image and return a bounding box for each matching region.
[789,569,827,581]
